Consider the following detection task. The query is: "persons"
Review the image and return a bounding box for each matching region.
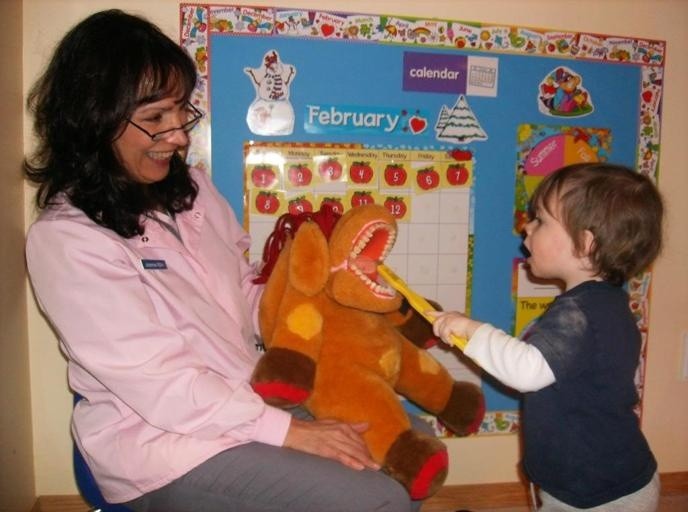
[423,161,665,510]
[19,8,427,512]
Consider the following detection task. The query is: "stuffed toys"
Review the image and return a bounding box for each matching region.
[247,206,483,500]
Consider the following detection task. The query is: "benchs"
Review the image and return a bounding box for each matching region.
[30,472,688,512]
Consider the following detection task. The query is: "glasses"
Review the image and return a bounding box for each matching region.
[120,99,206,144]
